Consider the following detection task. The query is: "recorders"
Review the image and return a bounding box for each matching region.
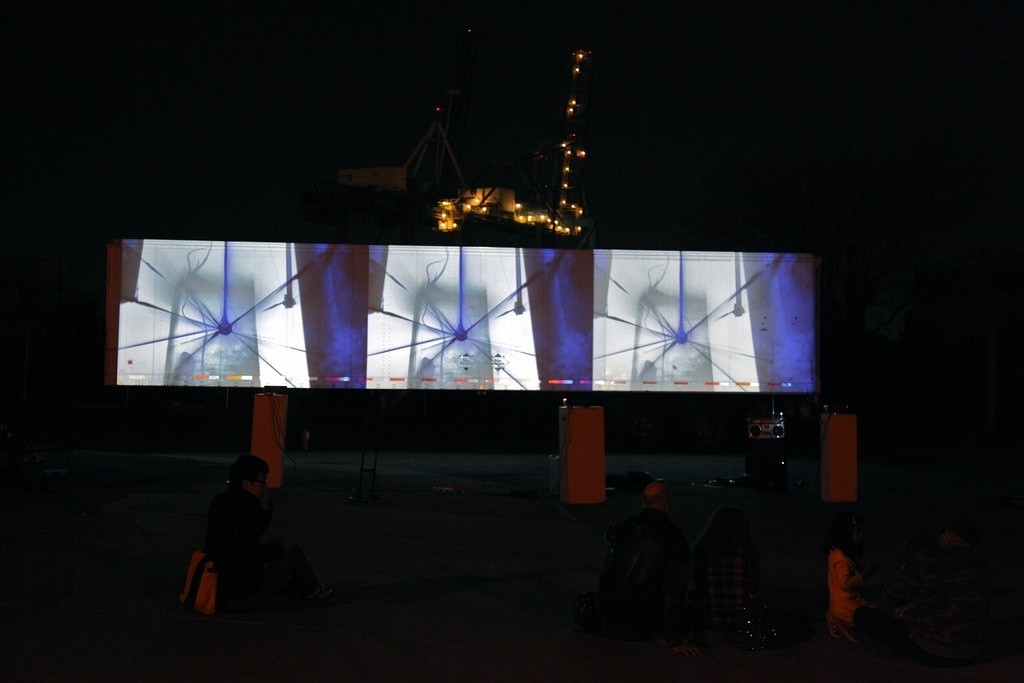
[746,411,786,439]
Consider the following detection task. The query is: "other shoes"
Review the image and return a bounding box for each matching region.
[837,620,862,644]
[826,610,840,639]
[306,585,334,600]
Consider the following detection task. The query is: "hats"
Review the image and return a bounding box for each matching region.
[644,481,670,503]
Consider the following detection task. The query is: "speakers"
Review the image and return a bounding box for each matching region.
[817,413,857,502]
[250,393,288,488]
[558,405,606,506]
[744,438,790,488]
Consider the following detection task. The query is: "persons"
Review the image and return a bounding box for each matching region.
[690,506,761,633]
[196,455,334,612]
[820,512,877,643]
[853,533,985,667]
[0,380,32,489]
[573,479,704,656]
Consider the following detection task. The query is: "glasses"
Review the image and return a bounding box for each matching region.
[249,479,265,485]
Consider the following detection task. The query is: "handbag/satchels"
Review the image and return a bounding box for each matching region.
[571,590,616,636]
[179,550,223,616]
[737,595,784,651]
